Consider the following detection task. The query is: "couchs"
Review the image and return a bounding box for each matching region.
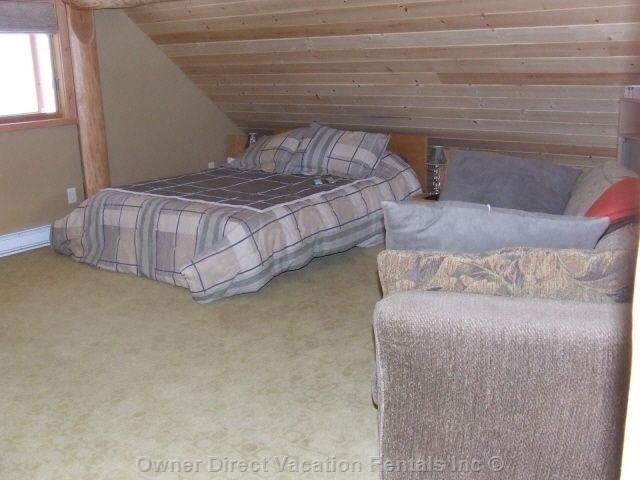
[370,153,640,480]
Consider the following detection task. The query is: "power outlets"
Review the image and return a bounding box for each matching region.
[209,162,215,169]
[66,188,78,204]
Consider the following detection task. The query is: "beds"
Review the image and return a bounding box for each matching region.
[50,127,428,304]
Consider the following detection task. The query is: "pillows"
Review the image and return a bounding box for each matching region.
[229,127,307,173]
[382,198,610,248]
[285,123,390,178]
[437,146,584,213]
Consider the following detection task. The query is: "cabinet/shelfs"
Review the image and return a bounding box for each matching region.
[617,97,640,169]
